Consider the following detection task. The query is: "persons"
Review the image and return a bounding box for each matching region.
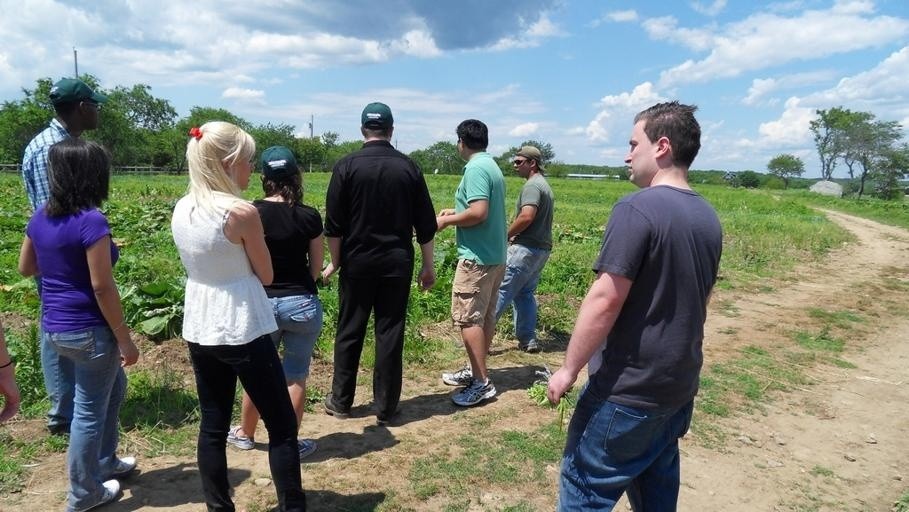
[226,144,325,459]
[18,138,141,512]
[171,120,308,510]
[436,115,510,407]
[23,78,111,433]
[544,99,726,512]
[497,145,553,354]
[0,320,24,427]
[322,102,438,425]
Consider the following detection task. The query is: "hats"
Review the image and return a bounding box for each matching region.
[261,145,296,176]
[362,103,393,130]
[516,146,546,171]
[49,79,107,105]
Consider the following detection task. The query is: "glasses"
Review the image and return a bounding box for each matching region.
[84,102,102,111]
[513,161,529,164]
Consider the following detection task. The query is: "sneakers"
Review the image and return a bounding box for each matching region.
[298,439,316,459]
[442,365,475,386]
[113,457,136,479]
[226,426,254,450]
[69,480,120,512]
[518,339,537,353]
[451,377,496,407]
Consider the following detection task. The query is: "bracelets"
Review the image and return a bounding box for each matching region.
[0,361,14,368]
[111,320,127,332]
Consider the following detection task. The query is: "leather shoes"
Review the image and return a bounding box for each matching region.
[324,398,350,419]
[376,405,402,426]
